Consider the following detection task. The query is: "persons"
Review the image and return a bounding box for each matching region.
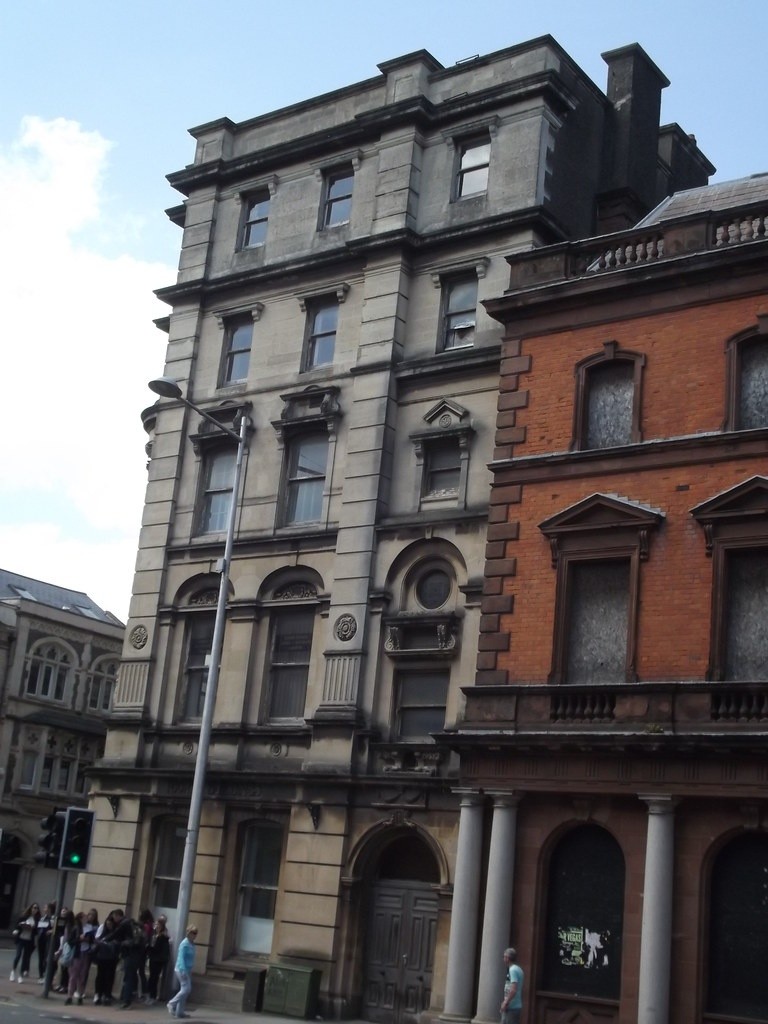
[498,948,525,1024]
[8,901,170,1010]
[166,924,198,1018]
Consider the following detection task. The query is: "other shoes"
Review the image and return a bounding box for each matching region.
[78,996,83,1005]
[64,996,72,1005]
[176,1014,190,1019]
[167,1002,175,1017]
[144,998,157,1006]
[17,976,23,984]
[55,985,68,993]
[94,994,136,1009]
[23,970,27,977]
[9,970,15,981]
[42,990,48,1000]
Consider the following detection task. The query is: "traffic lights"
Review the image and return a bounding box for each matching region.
[49,808,68,869]
[32,805,64,867]
[59,806,96,874]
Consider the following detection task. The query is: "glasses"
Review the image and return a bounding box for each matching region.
[143,920,149,925]
[193,932,197,935]
[34,906,39,910]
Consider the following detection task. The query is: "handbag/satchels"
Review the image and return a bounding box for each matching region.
[7,922,24,940]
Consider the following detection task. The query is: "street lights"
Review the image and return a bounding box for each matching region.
[147,377,250,1006]
